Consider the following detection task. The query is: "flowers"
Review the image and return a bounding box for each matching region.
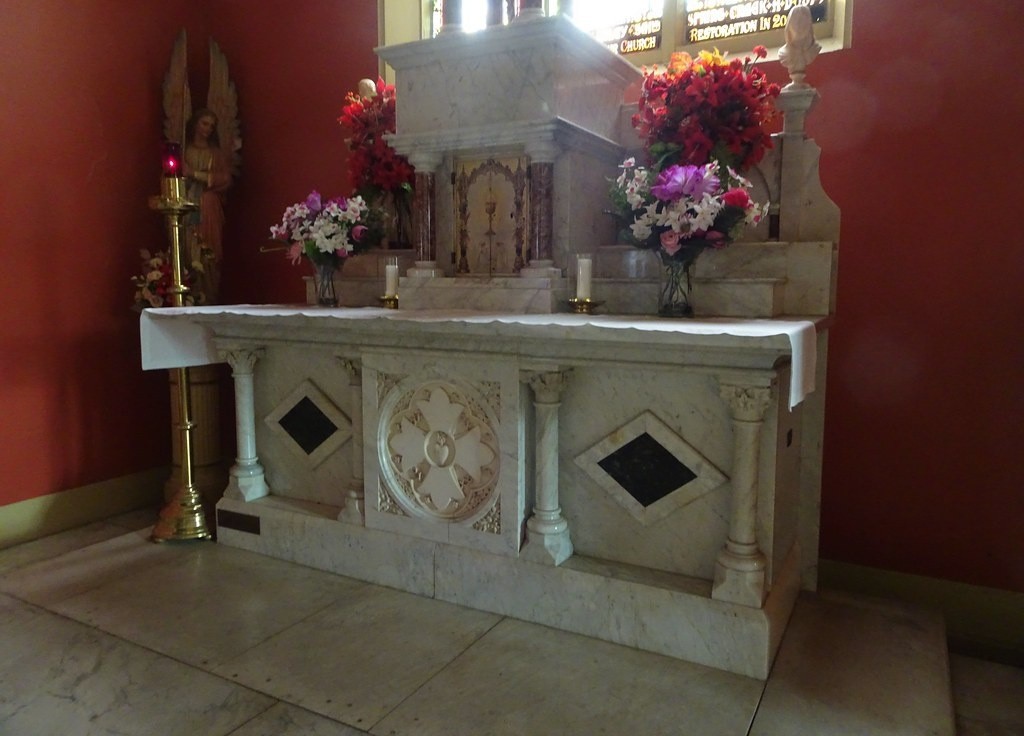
[337,76,416,243]
[632,46,781,176]
[270,190,369,300]
[132,234,214,308]
[605,157,770,311]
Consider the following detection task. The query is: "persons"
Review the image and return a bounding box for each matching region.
[778,2,822,75]
[166,108,237,304]
[358,79,377,101]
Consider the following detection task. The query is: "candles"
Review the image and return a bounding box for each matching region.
[577,258,592,299]
[386,265,399,297]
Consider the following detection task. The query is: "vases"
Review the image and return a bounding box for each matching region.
[385,193,413,249]
[315,266,343,307]
[656,249,696,319]
